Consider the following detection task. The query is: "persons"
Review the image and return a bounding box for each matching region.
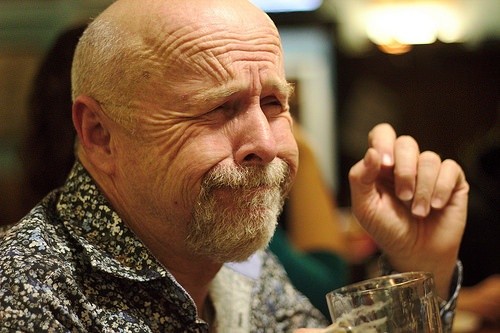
[0,1,469,333]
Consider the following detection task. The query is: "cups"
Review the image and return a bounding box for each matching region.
[325,271,443,333]
